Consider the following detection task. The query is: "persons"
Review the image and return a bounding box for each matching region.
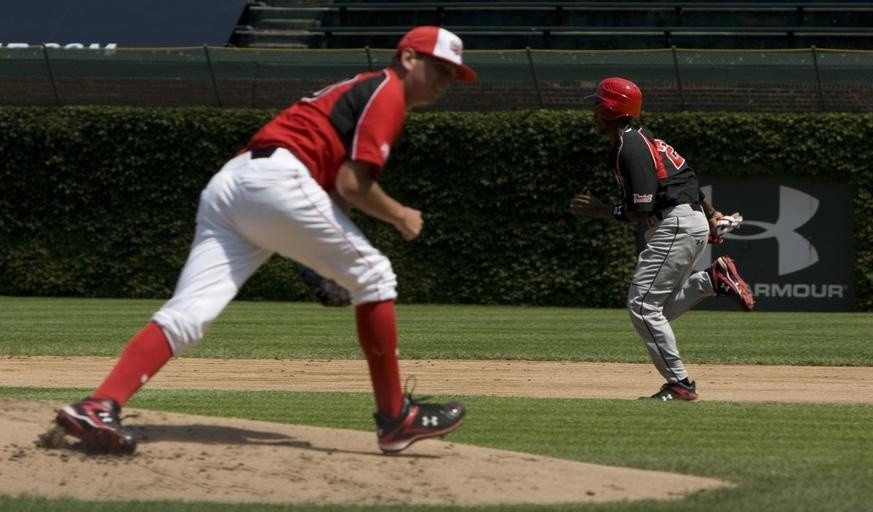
[55,25,478,453]
[568,77,754,402]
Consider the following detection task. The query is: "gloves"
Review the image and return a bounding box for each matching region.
[708,211,744,245]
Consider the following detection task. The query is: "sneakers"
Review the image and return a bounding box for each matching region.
[56,396,136,457]
[373,374,467,452]
[638,381,698,402]
[703,255,756,312]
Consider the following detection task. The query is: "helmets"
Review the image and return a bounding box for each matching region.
[583,77,642,121]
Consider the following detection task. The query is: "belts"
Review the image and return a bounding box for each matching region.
[645,202,702,230]
[251,145,278,159]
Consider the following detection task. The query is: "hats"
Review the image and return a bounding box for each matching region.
[397,26,477,83]
